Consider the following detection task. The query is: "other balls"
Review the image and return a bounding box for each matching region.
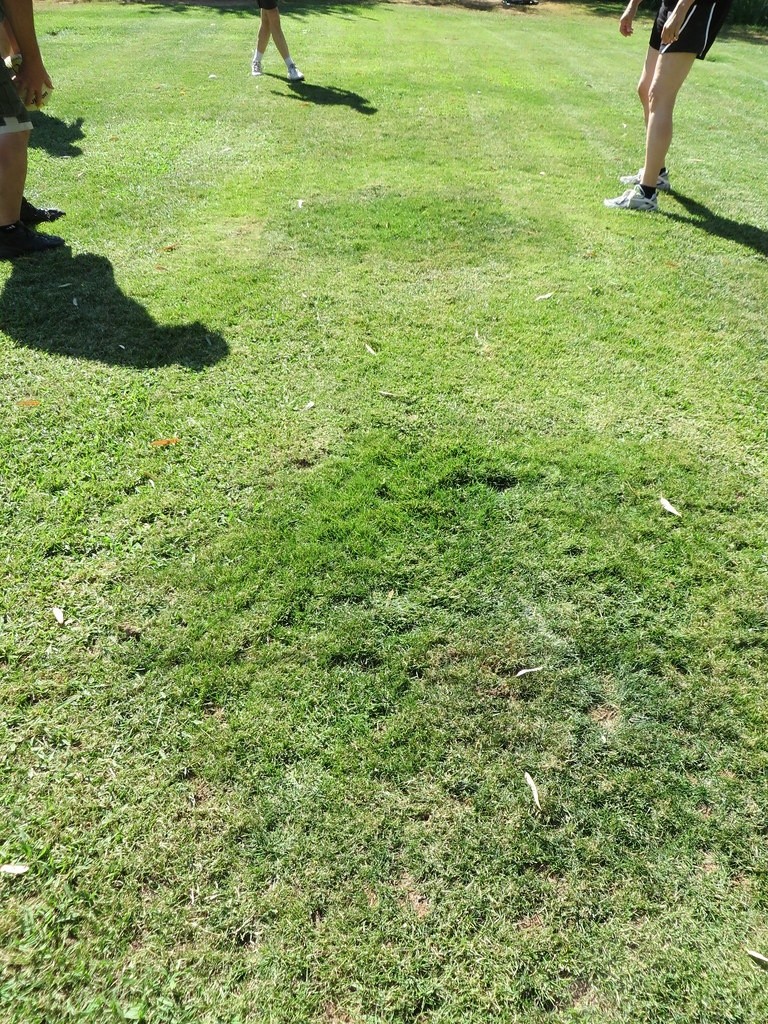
[3,53,52,111]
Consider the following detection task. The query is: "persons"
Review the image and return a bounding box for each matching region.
[250,0,305,81]
[604,0,733,210]
[0,0,63,256]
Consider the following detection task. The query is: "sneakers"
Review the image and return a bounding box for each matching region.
[0,220,64,257]
[287,63,304,80]
[20,197,66,225]
[621,167,670,189]
[251,59,263,75]
[603,185,660,210]
